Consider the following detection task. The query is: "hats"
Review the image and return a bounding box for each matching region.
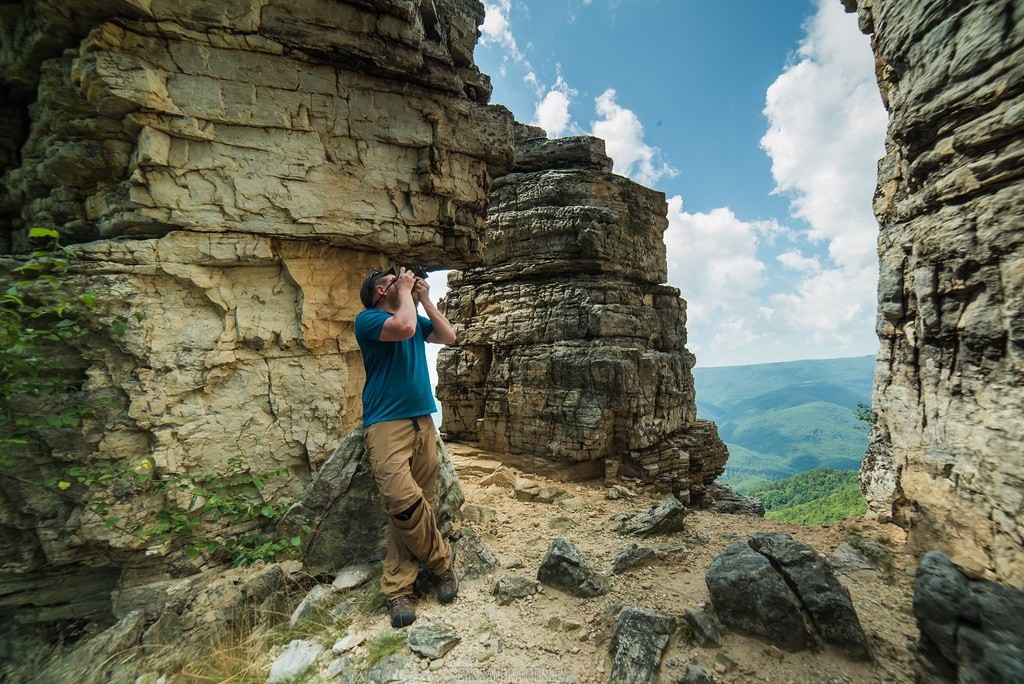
[360,266,396,309]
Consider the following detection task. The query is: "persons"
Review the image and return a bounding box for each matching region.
[352,264,459,629]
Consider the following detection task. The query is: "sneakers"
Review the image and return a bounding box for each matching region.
[389,594,416,628]
[433,561,459,602]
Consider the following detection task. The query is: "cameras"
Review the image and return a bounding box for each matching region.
[397,264,429,291]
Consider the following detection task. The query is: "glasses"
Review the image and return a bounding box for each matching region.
[365,269,382,295]
